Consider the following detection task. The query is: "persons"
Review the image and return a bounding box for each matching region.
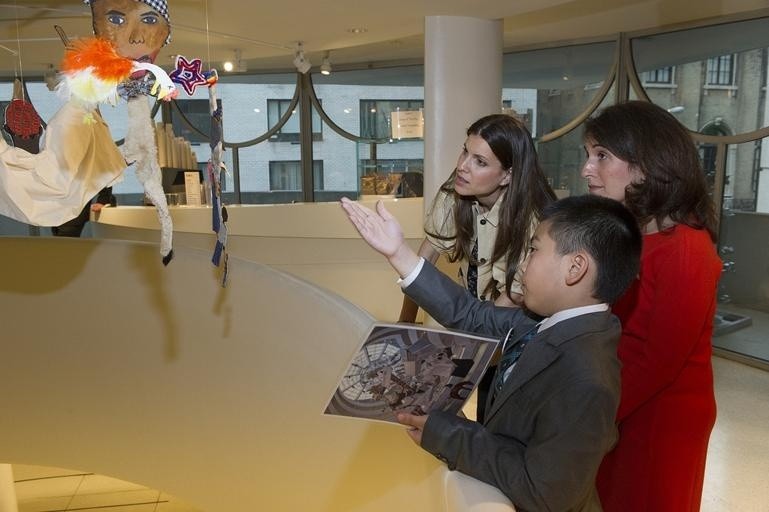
[578,101,723,511]
[340,191,643,511]
[394,113,559,328]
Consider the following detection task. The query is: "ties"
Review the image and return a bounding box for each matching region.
[494,324,542,401]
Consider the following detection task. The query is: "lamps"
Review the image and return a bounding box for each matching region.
[222,40,332,76]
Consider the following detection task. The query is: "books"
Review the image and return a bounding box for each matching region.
[321,321,502,429]
